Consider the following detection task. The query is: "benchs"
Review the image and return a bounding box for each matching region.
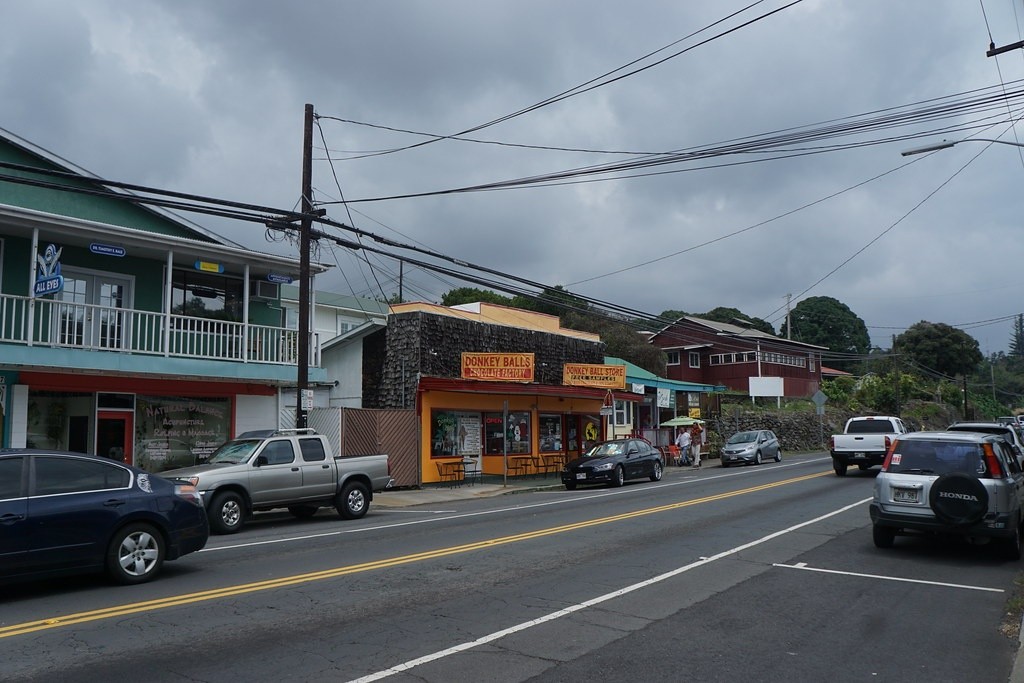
[690,451,710,460]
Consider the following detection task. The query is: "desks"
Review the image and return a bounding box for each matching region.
[443,462,475,488]
[544,455,572,478]
[512,457,540,480]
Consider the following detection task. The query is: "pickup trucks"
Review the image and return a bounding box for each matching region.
[153,428,393,535]
[828,416,910,476]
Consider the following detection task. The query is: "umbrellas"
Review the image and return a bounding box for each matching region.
[659,415,706,426]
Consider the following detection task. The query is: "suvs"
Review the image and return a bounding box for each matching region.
[870,432,1024,559]
[945,421,1024,473]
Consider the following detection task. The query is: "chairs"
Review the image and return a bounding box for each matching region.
[911,448,945,474]
[963,452,983,477]
[507,455,557,480]
[436,458,482,489]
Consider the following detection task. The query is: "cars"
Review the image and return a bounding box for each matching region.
[561,438,664,490]
[996,414,1024,446]
[0,449,209,588]
[719,429,782,466]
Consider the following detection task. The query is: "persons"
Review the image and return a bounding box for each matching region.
[690,422,704,468]
[675,427,692,467]
